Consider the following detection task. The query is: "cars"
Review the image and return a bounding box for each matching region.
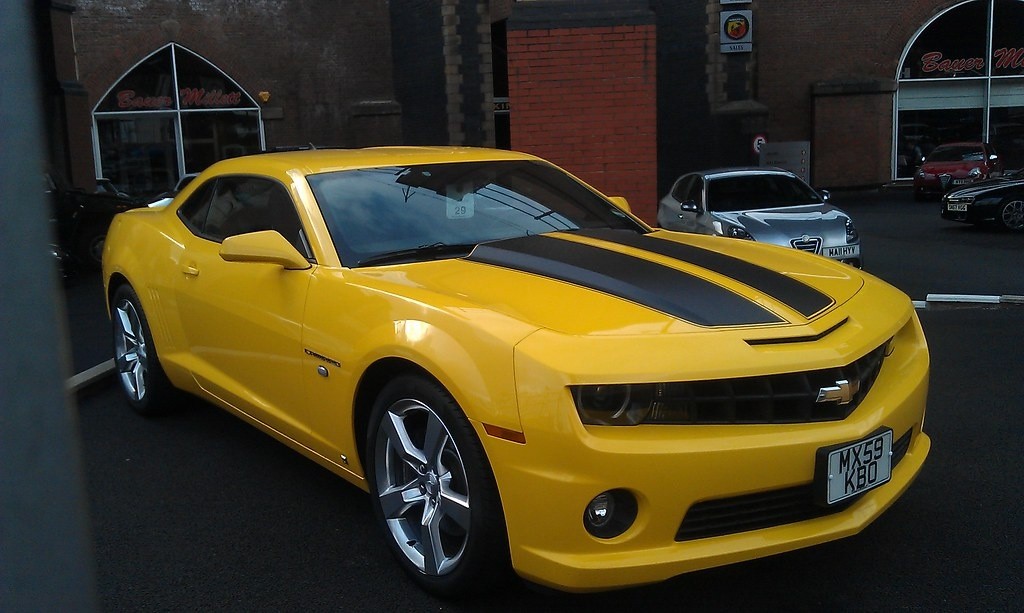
[658,166,864,276]
[940,165,1024,234]
[913,140,1004,199]
[44,171,197,280]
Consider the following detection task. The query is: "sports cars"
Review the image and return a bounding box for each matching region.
[101,144,933,607]
